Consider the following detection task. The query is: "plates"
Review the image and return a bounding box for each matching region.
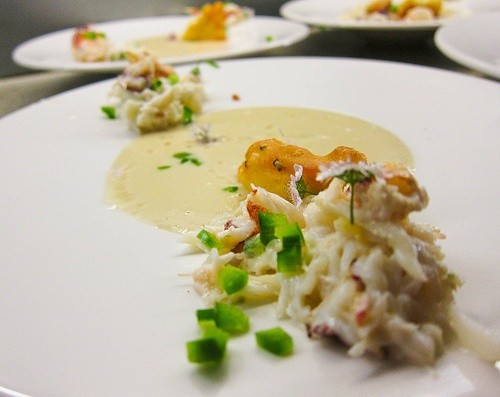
[432,1,500,78]
[280,0,474,30]
[0,55,500,397]
[10,11,313,70]
